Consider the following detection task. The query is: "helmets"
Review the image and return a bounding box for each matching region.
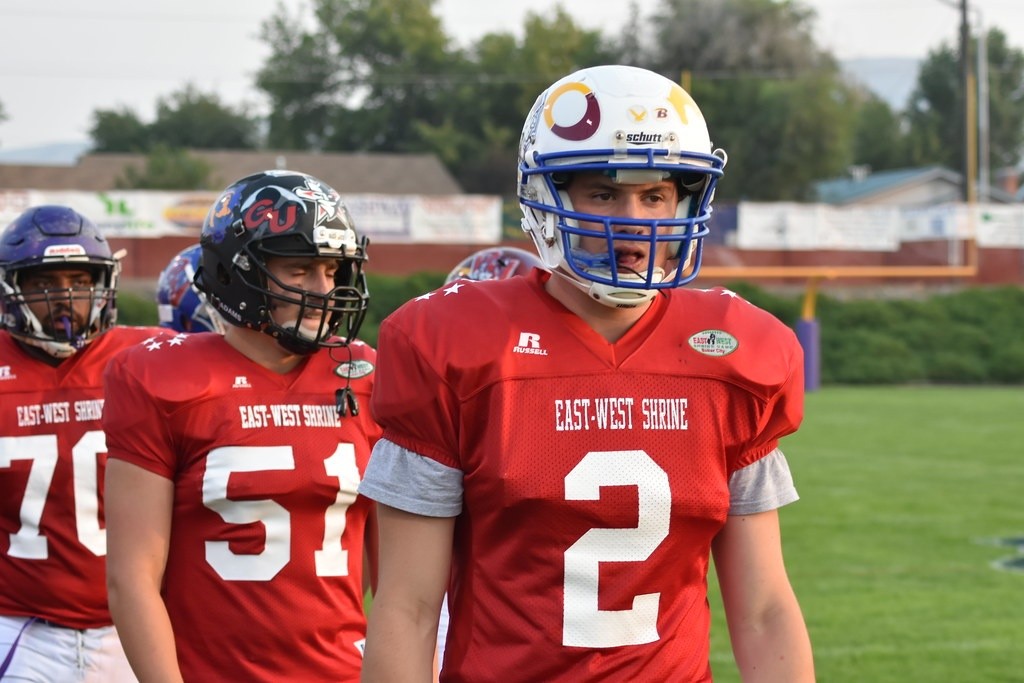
[444,246,545,287]
[0,205,118,358]
[200,169,365,331]
[155,243,223,333]
[516,65,715,271]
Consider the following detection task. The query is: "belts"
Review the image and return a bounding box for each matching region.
[31,616,69,629]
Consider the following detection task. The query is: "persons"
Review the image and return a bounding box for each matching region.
[0,65,817,683]
